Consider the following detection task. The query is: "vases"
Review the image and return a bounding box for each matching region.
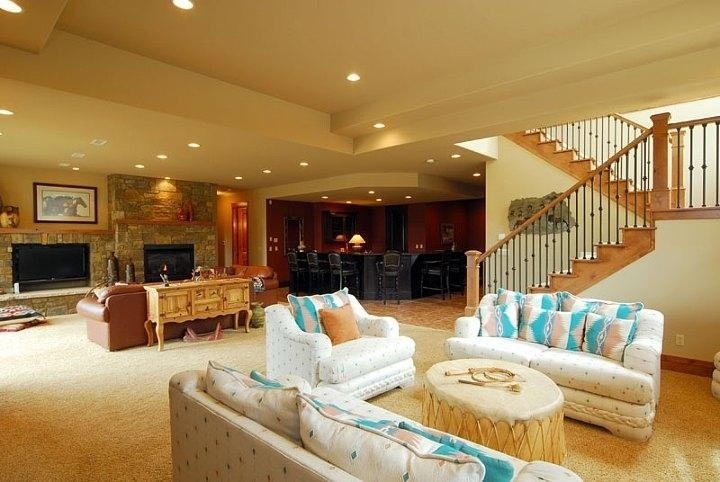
[107,252,119,287]
[188,202,194,221]
[125,258,135,283]
[177,208,187,221]
[251,302,264,328]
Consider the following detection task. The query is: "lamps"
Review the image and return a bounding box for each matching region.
[349,235,365,252]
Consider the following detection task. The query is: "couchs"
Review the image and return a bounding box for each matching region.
[76,265,279,352]
[168,370,582,482]
[448,294,664,442]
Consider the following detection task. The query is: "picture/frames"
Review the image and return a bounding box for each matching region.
[33,182,97,223]
[284,216,304,256]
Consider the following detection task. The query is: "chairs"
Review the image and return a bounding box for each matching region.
[265,294,415,400]
[287,250,466,304]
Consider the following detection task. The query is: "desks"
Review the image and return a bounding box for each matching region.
[144,278,251,351]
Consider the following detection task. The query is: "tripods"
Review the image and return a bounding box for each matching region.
[12,243,90,285]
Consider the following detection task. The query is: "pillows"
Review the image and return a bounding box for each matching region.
[561,292,643,320]
[495,289,561,324]
[581,313,637,360]
[518,306,586,352]
[318,303,360,346]
[477,303,518,339]
[287,287,348,333]
